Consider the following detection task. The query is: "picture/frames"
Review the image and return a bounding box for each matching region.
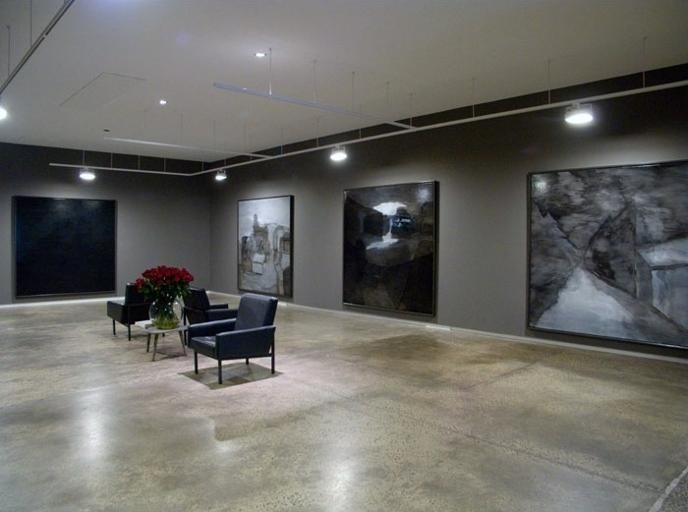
[526,159,688,356]
[237,195,294,299]
[342,180,440,318]
[11,195,118,299]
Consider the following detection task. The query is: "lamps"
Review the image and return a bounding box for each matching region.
[79,167,96,182]
[329,143,349,165]
[564,102,596,129]
[215,168,227,181]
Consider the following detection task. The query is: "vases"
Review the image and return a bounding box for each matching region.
[149,298,182,330]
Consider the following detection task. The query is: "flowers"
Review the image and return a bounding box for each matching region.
[133,265,195,330]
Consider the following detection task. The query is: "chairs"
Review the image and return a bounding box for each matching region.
[188,293,279,385]
[184,288,239,345]
[107,283,151,341]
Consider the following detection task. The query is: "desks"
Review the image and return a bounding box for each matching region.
[144,324,188,361]
